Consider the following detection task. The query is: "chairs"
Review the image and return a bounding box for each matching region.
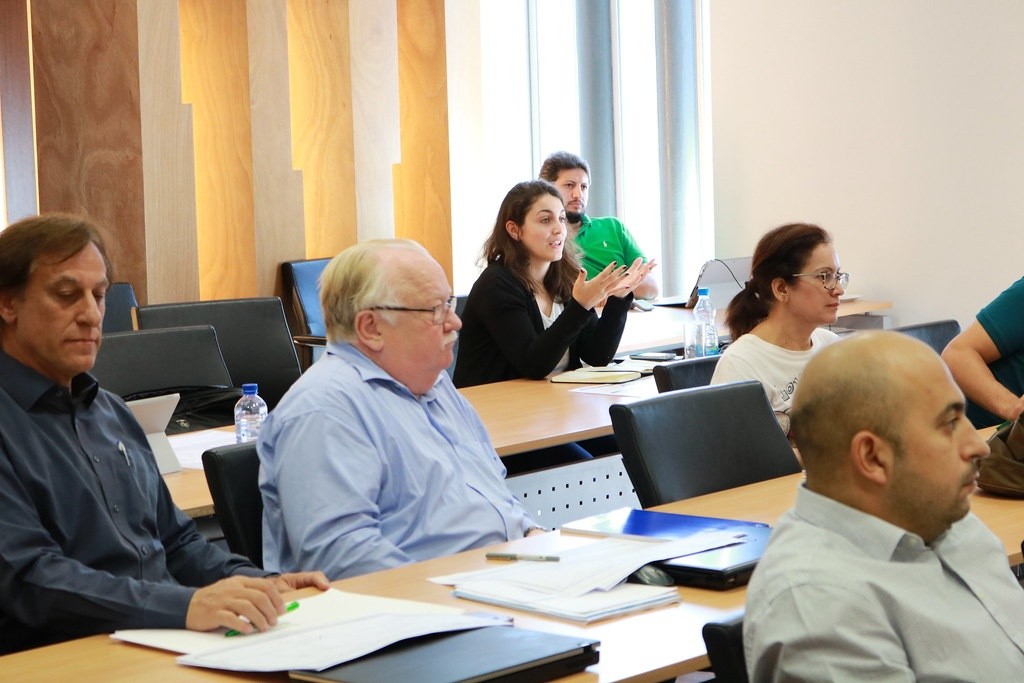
[84,325,233,400]
[701,608,750,683]
[652,355,721,393]
[101,281,136,333]
[282,257,330,371]
[888,319,962,355]
[203,438,262,567]
[607,382,801,509]
[128,295,301,413]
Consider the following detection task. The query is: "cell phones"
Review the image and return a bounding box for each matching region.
[630,351,677,360]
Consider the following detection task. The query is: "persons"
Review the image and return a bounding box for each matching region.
[538,152,658,312]
[0,213,334,659]
[941,277,1024,431]
[257,238,549,583]
[451,181,658,478]
[742,328,1024,683]
[709,223,846,469]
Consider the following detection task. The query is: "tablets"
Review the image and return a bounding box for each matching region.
[685,256,753,308]
[124,392,181,436]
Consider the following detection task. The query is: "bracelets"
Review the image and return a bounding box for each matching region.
[524,525,548,537]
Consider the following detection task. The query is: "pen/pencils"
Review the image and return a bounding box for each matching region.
[225,602,299,638]
[486,554,559,562]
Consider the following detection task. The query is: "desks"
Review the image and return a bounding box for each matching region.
[140,349,707,521]
[0,439,1024,683]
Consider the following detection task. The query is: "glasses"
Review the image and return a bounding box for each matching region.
[362,295,456,323]
[789,272,850,290]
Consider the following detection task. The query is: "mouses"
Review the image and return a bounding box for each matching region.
[628,300,654,313]
[626,564,674,587]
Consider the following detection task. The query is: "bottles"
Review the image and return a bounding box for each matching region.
[693,287,720,357]
[233,383,269,443]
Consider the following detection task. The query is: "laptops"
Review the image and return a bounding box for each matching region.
[288,627,600,683]
[561,507,775,591]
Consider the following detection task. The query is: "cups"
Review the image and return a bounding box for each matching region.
[683,320,706,359]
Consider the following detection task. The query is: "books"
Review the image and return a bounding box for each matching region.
[560,506,767,547]
[550,358,683,384]
